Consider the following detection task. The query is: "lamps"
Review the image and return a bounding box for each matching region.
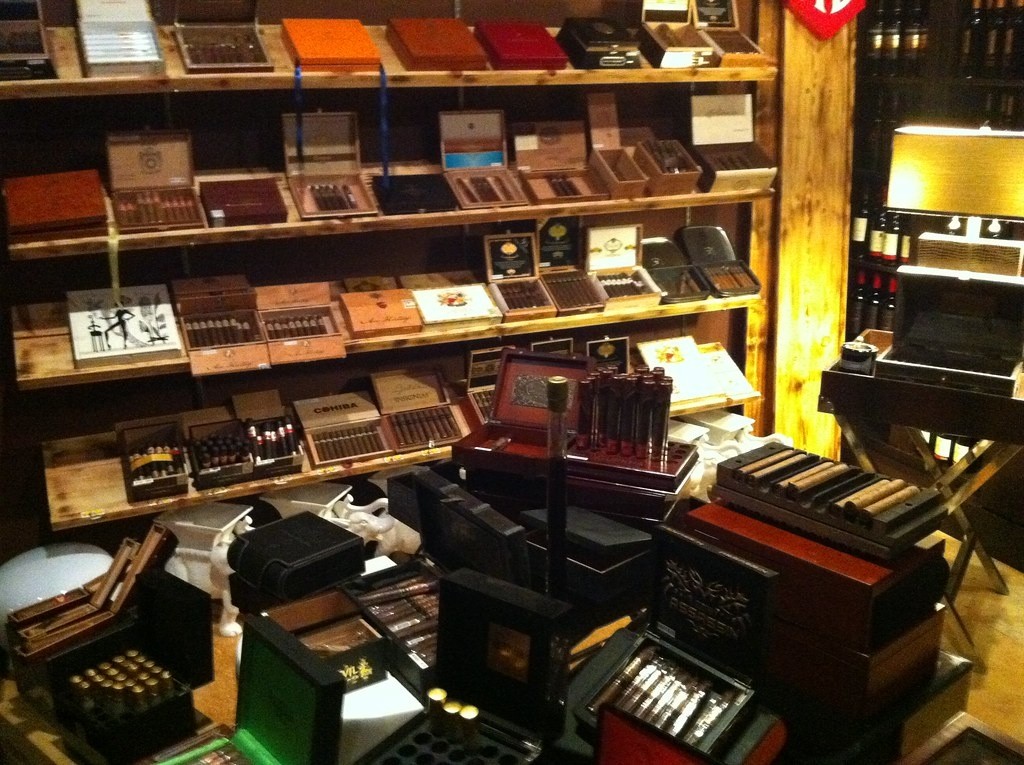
[885,124,1024,238]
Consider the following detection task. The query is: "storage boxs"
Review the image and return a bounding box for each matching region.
[0,2,1024,765]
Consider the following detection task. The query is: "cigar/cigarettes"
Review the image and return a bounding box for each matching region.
[586,644,738,748]
[185,742,252,765]
[734,448,921,531]
[296,576,441,668]
[83,23,778,504]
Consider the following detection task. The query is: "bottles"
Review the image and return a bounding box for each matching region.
[918,429,983,474]
[864,0,927,78]
[880,279,897,332]
[864,91,902,170]
[957,0,1024,81]
[847,272,866,341]
[978,90,1018,132]
[863,275,882,332]
[851,184,911,266]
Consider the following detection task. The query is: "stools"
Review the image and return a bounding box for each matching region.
[812,393,1024,657]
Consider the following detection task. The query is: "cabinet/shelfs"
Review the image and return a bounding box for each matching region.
[847,75,1024,276]
[1,1,781,532]
